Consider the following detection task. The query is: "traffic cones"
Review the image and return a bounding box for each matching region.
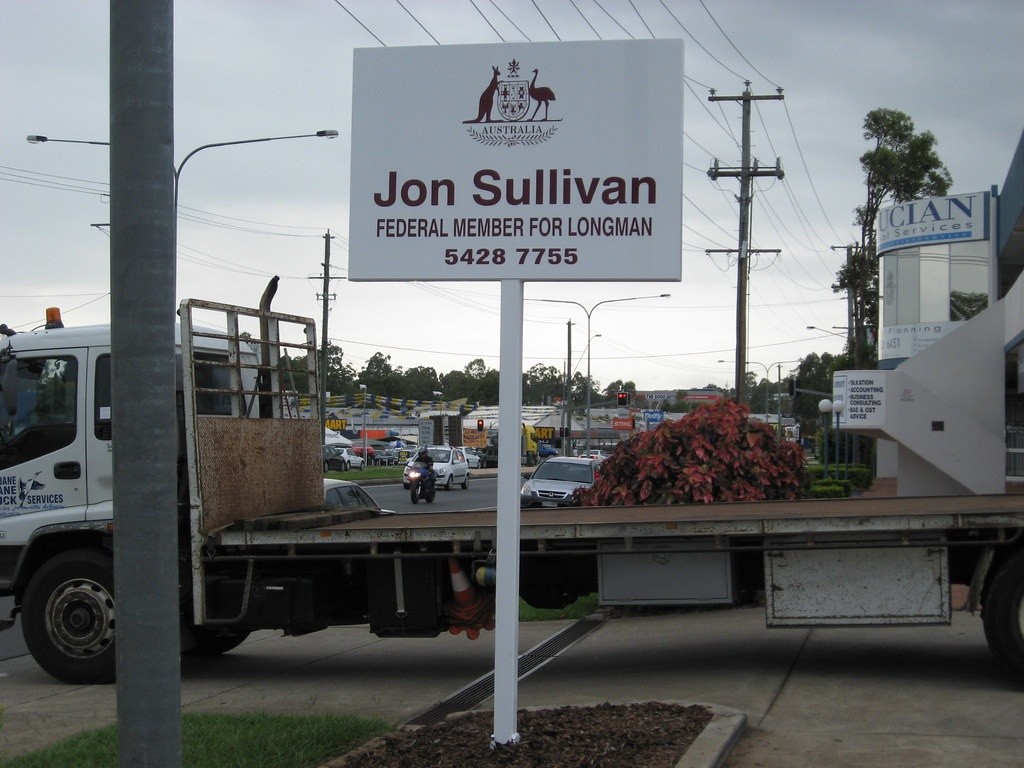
[441,557,495,627]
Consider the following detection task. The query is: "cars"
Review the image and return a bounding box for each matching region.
[520,455,609,510]
[537,445,558,458]
[398,446,470,491]
[578,449,605,460]
[323,439,487,474]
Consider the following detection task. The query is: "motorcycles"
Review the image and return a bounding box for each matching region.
[402,460,437,503]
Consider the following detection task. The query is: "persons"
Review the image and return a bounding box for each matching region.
[414,448,434,493]
[573,447,578,457]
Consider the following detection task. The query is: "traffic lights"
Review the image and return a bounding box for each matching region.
[565,427,570,437]
[616,392,628,406]
[560,427,564,437]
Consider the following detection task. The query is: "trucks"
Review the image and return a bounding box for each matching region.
[1,293,1024,690]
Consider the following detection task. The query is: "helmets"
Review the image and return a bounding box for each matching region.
[418,445,428,454]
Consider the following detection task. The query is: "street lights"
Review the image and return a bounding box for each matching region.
[525,294,673,459]
[744,358,800,426]
[24,129,339,255]
